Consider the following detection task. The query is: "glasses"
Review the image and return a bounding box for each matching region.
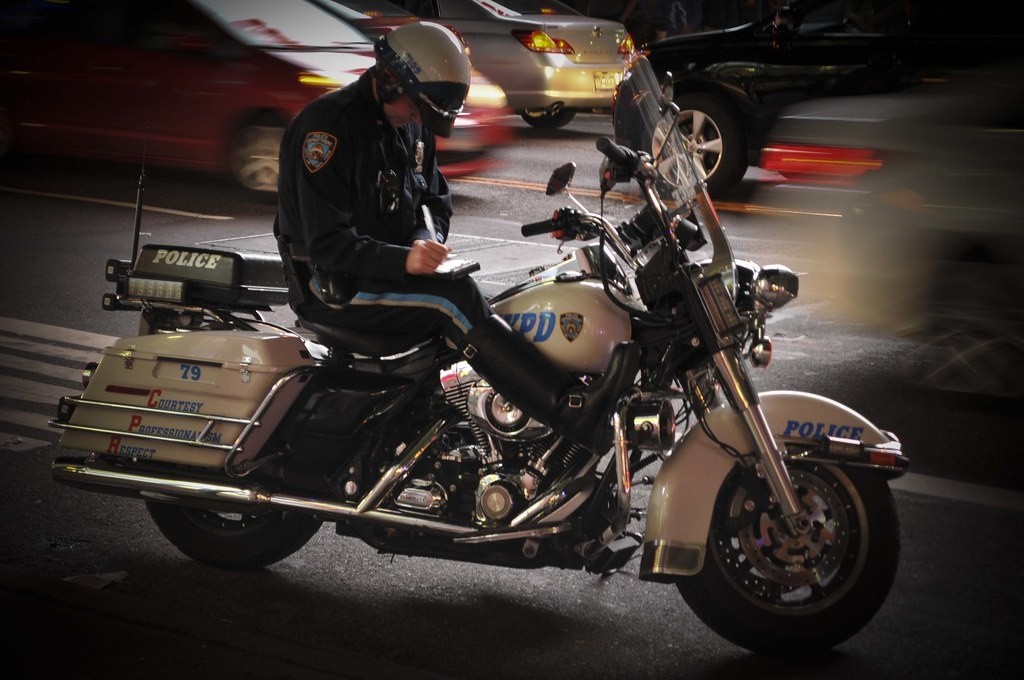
[377,168,400,220]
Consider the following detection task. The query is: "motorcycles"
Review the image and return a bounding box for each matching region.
[53,45,906,663]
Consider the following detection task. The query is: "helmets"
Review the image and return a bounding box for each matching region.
[374,21,471,138]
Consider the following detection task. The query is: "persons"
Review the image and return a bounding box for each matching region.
[274,20,637,455]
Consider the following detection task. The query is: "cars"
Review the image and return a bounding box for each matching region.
[611,0,1023,217]
[333,0,641,131]
[755,49,1024,430]
[0,1,514,208]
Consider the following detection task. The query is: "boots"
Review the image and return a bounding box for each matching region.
[458,314,640,456]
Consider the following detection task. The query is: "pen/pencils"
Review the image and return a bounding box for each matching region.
[422,205,443,266]
